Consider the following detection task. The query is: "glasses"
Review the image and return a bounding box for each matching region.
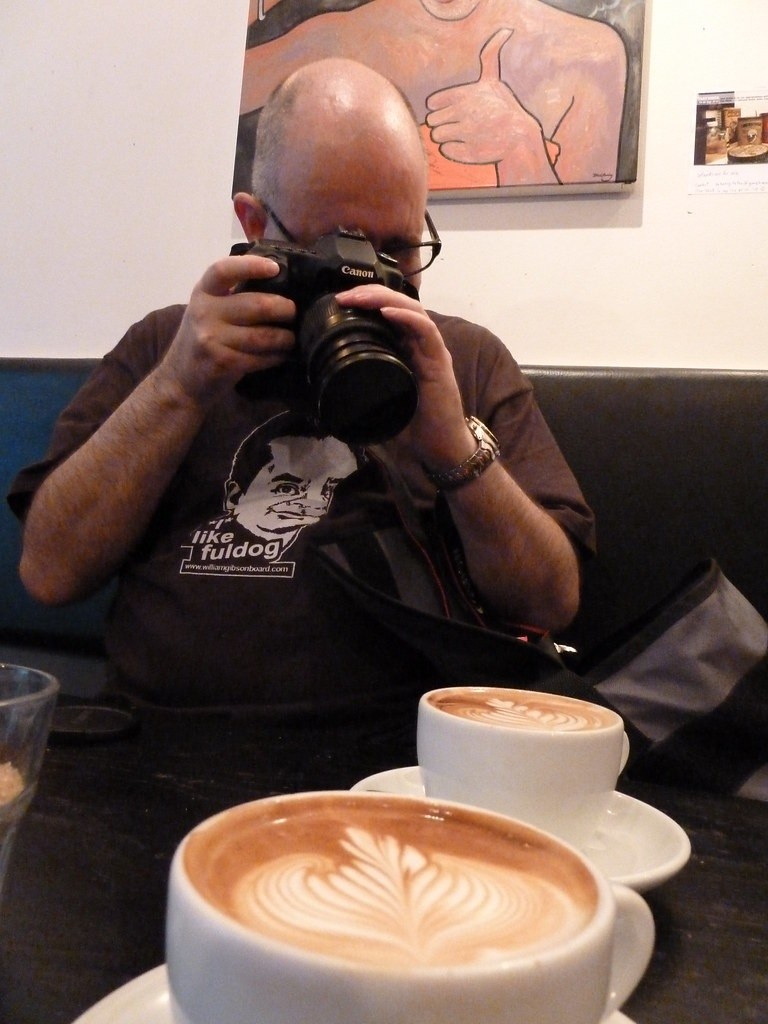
[258,197,441,279]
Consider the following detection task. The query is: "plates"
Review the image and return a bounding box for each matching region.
[73,962,640,1024]
[351,766,692,886]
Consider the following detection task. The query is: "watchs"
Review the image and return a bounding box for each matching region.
[420,415,503,492]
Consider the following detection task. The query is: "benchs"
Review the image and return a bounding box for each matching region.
[2,359,768,802]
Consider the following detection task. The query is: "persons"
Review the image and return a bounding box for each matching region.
[0,55,598,1023]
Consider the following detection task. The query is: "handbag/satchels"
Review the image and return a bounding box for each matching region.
[568,556,768,795]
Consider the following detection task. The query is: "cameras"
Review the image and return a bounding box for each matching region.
[226,222,420,446]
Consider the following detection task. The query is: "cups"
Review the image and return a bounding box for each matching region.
[0,661,60,902]
[419,688,631,856]
[164,793,655,1024]
